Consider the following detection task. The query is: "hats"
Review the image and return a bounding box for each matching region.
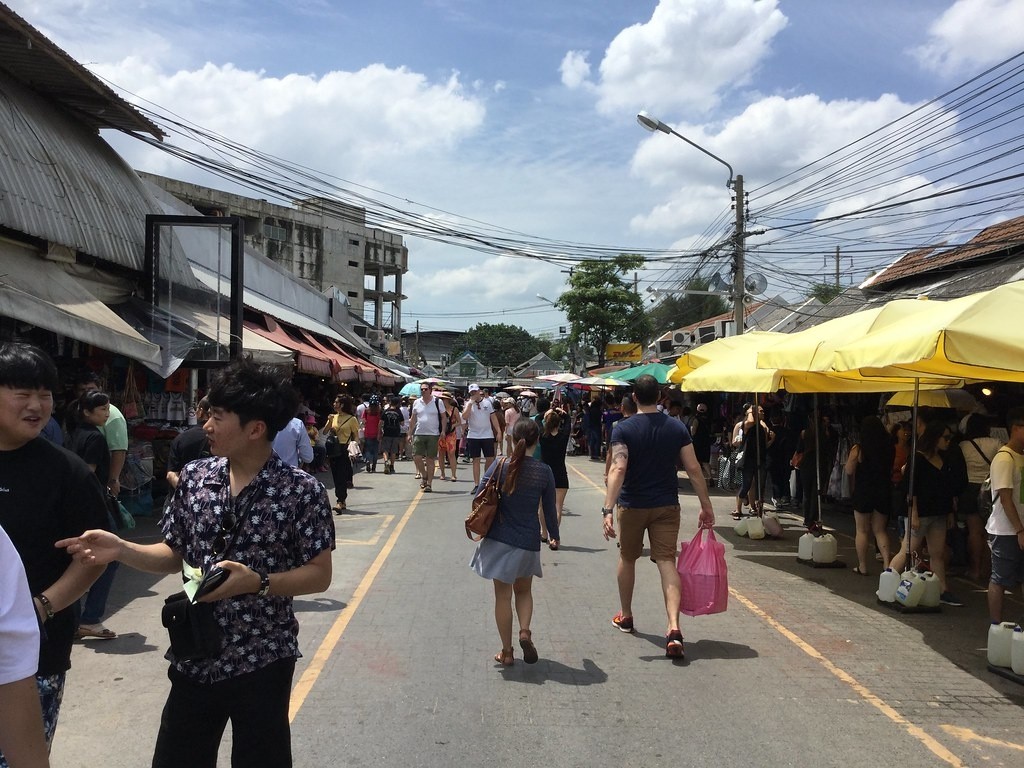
[501,397,516,405]
[369,394,380,406]
[467,383,480,394]
[696,402,708,413]
[305,416,317,424]
[436,391,452,400]
[409,393,417,400]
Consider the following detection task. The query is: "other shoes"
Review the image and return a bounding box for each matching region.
[330,498,347,512]
[366,459,457,492]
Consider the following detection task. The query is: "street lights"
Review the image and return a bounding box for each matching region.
[637,111,745,335]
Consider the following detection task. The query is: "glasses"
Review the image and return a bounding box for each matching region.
[207,512,238,559]
[420,388,428,392]
[940,434,952,441]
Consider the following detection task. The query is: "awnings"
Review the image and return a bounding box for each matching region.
[0,239,427,386]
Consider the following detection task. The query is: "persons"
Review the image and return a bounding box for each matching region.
[473,418,560,669]
[55,347,334,768]
[601,374,715,658]
[0,342,119,768]
[38,358,1024,636]
[0,526,51,768]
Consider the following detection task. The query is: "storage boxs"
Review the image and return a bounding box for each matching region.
[140,455,154,478]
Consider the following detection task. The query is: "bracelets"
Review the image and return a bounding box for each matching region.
[1016,528,1024,534]
[109,479,118,484]
[262,572,270,597]
[256,571,265,597]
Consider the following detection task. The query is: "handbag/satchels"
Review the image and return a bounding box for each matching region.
[161,590,222,665]
[463,457,508,542]
[717,442,887,505]
[435,398,452,435]
[113,497,137,538]
[675,523,729,617]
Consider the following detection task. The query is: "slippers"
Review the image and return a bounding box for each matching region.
[852,565,868,576]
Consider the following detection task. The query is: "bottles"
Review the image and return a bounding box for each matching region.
[987,621,1024,675]
[798,533,838,563]
[734,511,783,539]
[878,567,940,606]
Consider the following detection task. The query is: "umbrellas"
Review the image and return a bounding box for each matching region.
[399,281,1024,573]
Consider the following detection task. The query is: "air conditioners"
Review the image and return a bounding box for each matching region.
[655,339,674,357]
[351,323,369,338]
[366,330,385,344]
[715,319,735,338]
[672,330,691,346]
[694,325,716,344]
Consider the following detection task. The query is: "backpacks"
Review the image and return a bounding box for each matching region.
[383,410,401,437]
[975,476,1001,522]
[324,415,355,461]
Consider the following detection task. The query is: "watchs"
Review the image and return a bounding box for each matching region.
[35,594,55,618]
[602,507,613,515]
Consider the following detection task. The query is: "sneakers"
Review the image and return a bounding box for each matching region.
[664,629,686,659]
[611,611,635,633]
[939,590,964,608]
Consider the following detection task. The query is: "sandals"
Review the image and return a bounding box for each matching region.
[519,629,539,664]
[494,647,514,668]
[77,625,117,639]
